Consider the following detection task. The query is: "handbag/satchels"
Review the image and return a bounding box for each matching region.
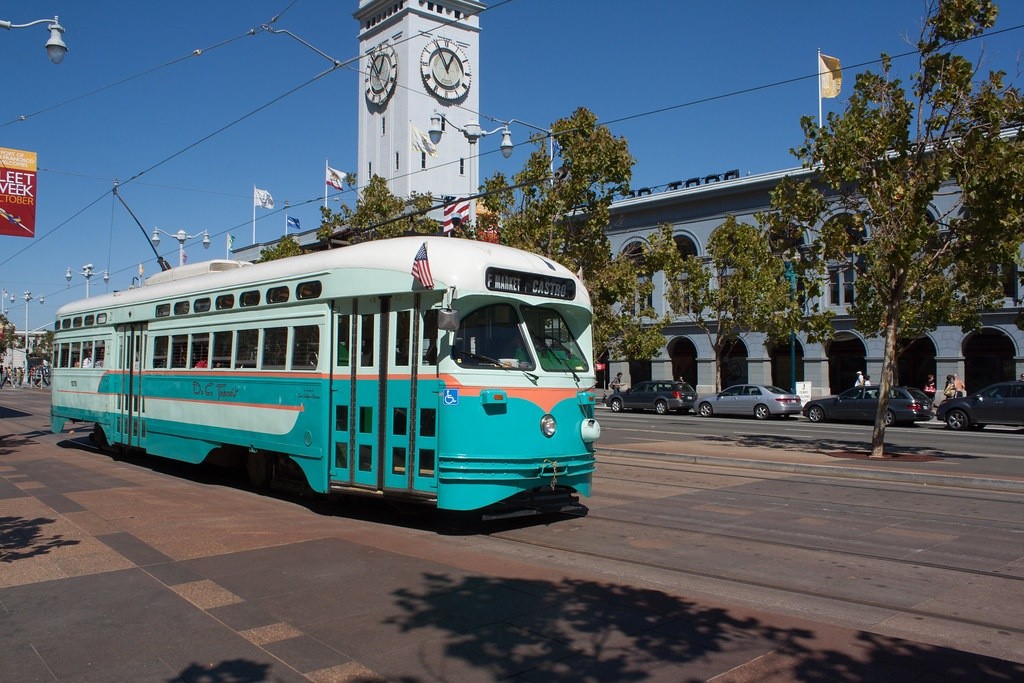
[944,384,954,396]
[609,381,614,389]
[925,386,935,392]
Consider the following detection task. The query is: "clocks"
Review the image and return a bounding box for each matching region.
[421,36,472,100]
[363,43,398,103]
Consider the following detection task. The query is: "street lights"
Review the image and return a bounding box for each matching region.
[152,226,210,267]
[429,109,513,238]
[11,291,45,383]
[782,250,801,393]
[66,264,109,298]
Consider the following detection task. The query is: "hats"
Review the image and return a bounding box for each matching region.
[857,371,862,373]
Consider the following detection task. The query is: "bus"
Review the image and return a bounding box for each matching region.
[51,235,602,522]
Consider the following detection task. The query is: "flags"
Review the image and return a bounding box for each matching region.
[182,251,187,263]
[326,166,347,191]
[226,233,235,253]
[441,194,470,234]
[408,124,437,159]
[286,216,300,229]
[139,264,144,278]
[412,243,434,290]
[818,54,842,98]
[254,189,275,209]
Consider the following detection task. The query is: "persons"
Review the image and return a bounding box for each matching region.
[74,349,104,368]
[678,376,683,382]
[855,371,872,399]
[611,373,625,394]
[0,365,51,388]
[925,374,966,404]
[1021,373,1024,381]
[506,315,562,363]
[154,344,318,368]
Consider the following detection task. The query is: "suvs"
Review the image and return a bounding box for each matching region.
[936,379,1024,431]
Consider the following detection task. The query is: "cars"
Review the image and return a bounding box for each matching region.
[606,380,698,414]
[801,385,934,428]
[692,384,803,419]
